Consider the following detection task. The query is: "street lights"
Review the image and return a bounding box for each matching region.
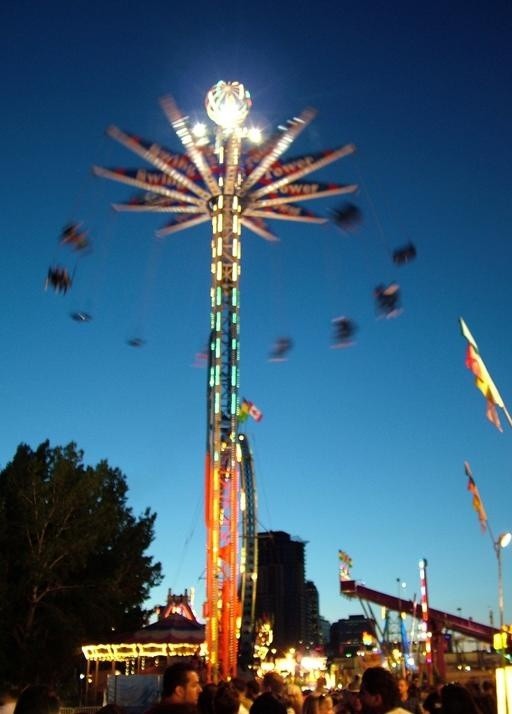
[495,533,511,713]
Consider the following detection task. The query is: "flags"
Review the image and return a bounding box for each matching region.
[240,397,250,416]
[459,316,506,434]
[338,547,352,567]
[464,463,487,534]
[236,404,247,423]
[248,401,262,422]
